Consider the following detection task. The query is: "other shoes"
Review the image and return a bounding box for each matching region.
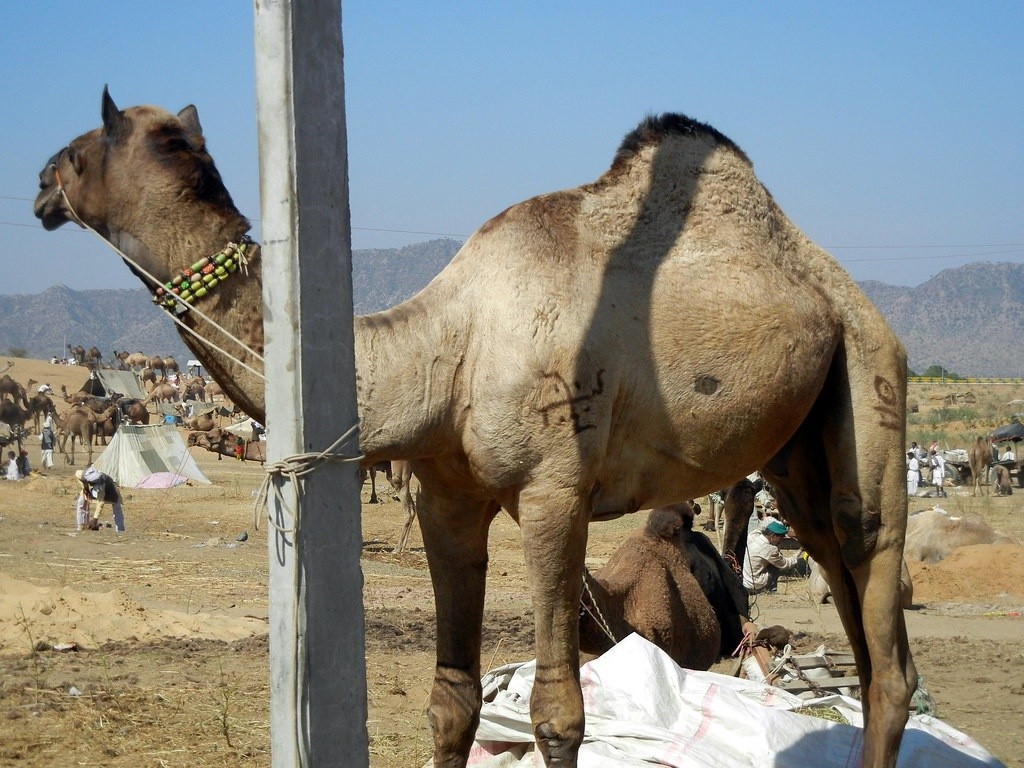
[77,525,85,531]
[772,584,777,591]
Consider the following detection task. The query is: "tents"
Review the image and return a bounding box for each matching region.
[78,369,150,401]
[91,424,211,489]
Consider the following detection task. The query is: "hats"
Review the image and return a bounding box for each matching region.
[20,450,28,456]
[85,467,105,484]
[766,521,788,534]
[43,422,51,428]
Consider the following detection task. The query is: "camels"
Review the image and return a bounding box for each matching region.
[968,430,1013,500]
[0,343,266,476]
[33,82,918,768]
[579,479,768,672]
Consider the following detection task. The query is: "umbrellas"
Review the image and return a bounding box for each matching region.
[988,422,1024,461]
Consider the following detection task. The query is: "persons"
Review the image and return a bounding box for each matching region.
[38,383,54,396]
[51,356,78,366]
[76,469,125,531]
[1001,446,1015,461]
[182,398,192,428]
[0,414,57,482]
[740,488,806,683]
[906,439,947,498]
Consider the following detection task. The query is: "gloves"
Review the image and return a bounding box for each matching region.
[88,518,98,530]
